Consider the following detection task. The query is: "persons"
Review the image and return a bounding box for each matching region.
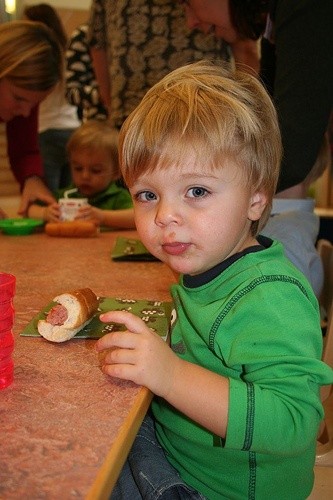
[66,23,108,124]
[87,1,258,131]
[26,122,138,229]
[179,1,333,118]
[95,58,332,500]
[257,74,325,302]
[0,19,66,234]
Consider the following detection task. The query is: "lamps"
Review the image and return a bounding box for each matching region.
[293,240,333,456]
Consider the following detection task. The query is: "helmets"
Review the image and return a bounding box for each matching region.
[1,218,44,235]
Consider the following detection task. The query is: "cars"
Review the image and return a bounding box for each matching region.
[59,198,88,222]
[0,272,16,391]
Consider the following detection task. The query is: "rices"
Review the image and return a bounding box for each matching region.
[37,288,99,343]
[45,221,100,239]
